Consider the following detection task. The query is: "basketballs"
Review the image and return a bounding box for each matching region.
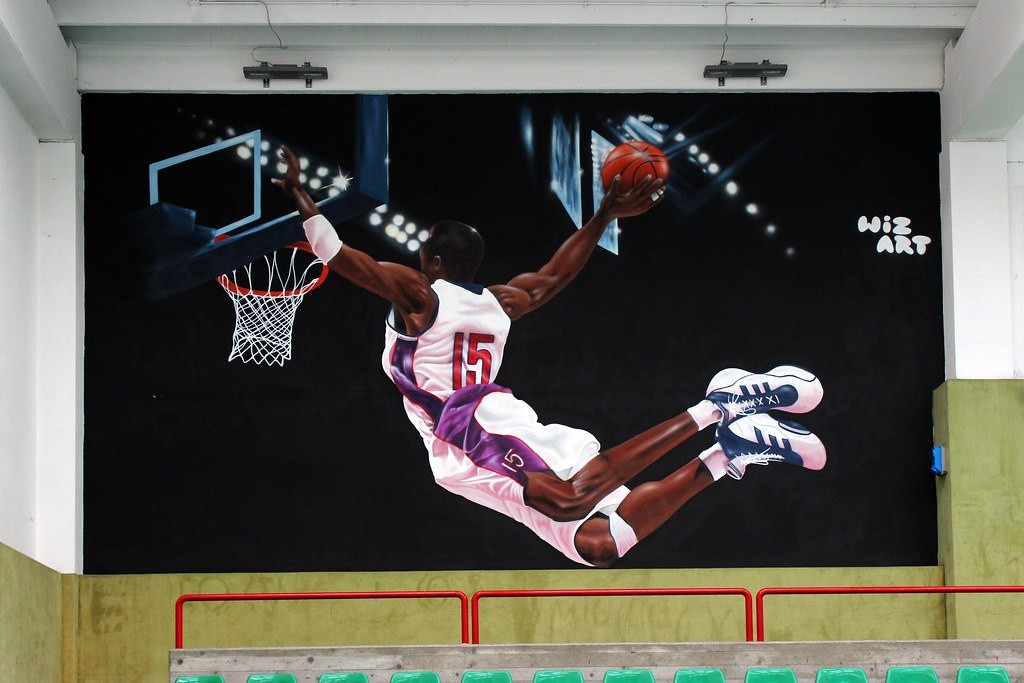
[600,140,671,195]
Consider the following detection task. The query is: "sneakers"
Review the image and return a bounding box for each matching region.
[714,411,827,480]
[706,366,824,424]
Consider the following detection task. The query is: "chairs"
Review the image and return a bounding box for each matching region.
[176,667,1016,683]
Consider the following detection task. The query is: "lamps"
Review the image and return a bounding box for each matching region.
[244,60,327,88]
[703,59,787,90]
